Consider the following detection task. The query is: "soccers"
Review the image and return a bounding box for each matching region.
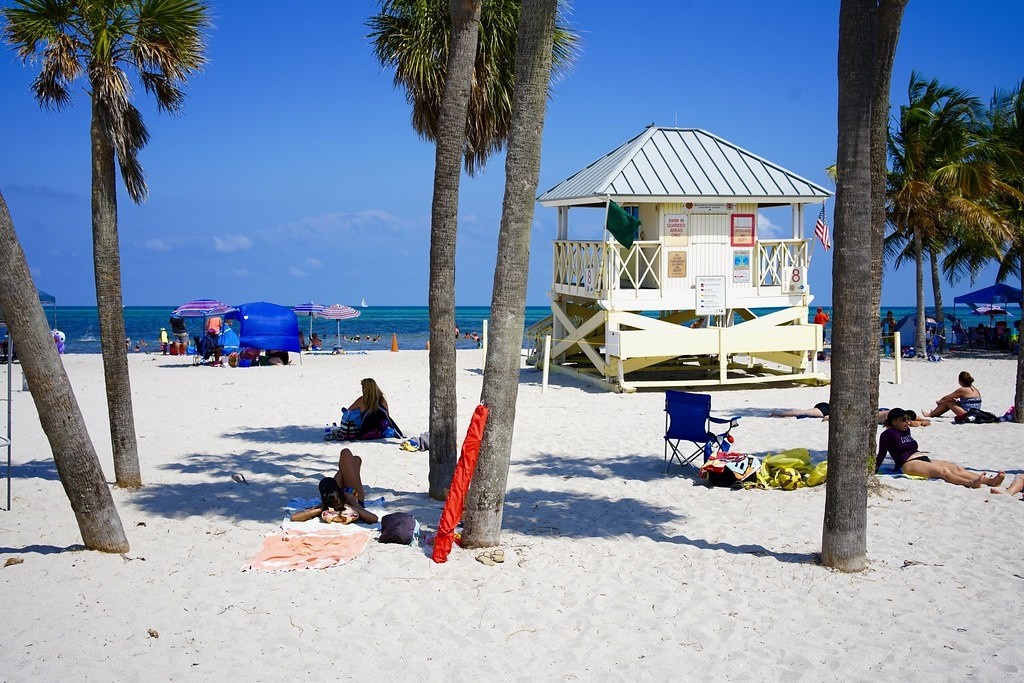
[55,330,65,343]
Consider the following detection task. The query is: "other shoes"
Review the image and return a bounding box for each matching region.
[177,353,184,356]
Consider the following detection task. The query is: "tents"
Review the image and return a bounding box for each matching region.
[35,289,55,332]
[951,283,1022,343]
[216,302,302,367]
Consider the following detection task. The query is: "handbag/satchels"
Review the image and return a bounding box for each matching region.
[339,420,359,440]
[342,407,362,430]
[1004,405,1016,422]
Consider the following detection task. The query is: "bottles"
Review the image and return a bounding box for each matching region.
[324,424,330,434]
[333,422,337,429]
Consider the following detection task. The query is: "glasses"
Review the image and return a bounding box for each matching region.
[896,417,909,422]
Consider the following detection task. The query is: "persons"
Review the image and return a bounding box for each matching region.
[921,371,981,417]
[240,347,289,365]
[335,334,380,343]
[456,326,482,349]
[814,307,830,343]
[126,336,147,351]
[201,316,222,362]
[881,311,896,346]
[170,307,188,355]
[769,403,930,427]
[990,473,1024,496]
[299,331,326,346]
[875,408,1006,489]
[348,378,388,434]
[290,449,378,524]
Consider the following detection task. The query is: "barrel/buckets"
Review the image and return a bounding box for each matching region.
[238,359,252,367]
[21,372,29,390]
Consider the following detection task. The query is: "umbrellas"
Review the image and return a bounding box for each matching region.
[318,304,361,349]
[971,305,1014,317]
[291,301,325,345]
[170,299,240,364]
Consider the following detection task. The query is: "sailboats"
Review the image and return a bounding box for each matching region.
[361,296,370,308]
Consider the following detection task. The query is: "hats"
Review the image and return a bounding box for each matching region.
[208,328,216,333]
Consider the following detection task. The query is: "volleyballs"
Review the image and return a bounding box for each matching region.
[778,468,802,490]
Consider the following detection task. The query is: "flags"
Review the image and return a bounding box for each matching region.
[815,204,831,251]
[607,200,642,250]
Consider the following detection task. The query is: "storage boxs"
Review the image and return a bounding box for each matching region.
[238,359,252,366]
[334,344,342,351]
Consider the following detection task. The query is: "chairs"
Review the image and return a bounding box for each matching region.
[663,389,741,476]
[193,335,225,366]
[902,327,1012,358]
